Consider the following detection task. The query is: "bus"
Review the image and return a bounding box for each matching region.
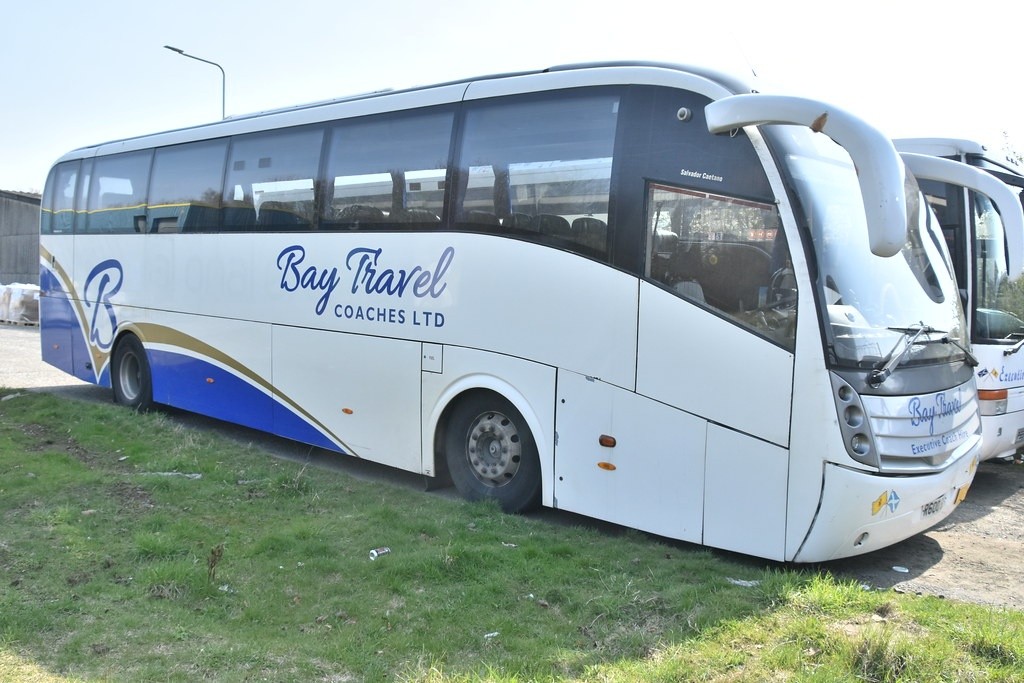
[38,62,1024,564]
[255,132,1024,464]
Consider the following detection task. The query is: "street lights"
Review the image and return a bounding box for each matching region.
[164,45,225,122]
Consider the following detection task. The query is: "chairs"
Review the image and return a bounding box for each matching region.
[530,215,570,235]
[502,213,533,230]
[572,218,606,252]
[350,204,385,222]
[463,210,500,225]
[653,230,679,285]
[398,208,441,223]
